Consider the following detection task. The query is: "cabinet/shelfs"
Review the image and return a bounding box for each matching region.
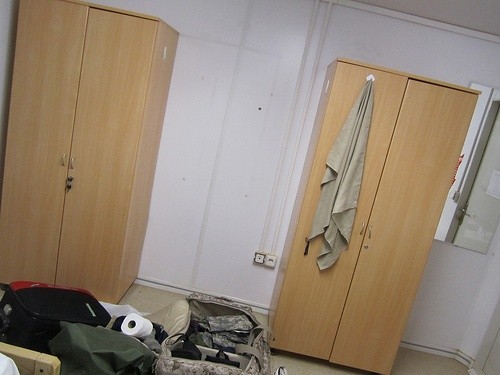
[268,56,481,374]
[1,0,181,304]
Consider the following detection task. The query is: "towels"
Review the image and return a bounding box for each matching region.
[305,79,376,272]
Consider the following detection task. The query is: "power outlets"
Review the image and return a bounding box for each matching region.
[254,252,266,264]
[265,254,277,267]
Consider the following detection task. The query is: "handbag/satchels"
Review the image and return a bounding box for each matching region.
[2,282,110,352]
[155,293,272,375]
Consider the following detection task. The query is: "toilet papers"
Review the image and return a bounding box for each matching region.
[120,313,153,339]
